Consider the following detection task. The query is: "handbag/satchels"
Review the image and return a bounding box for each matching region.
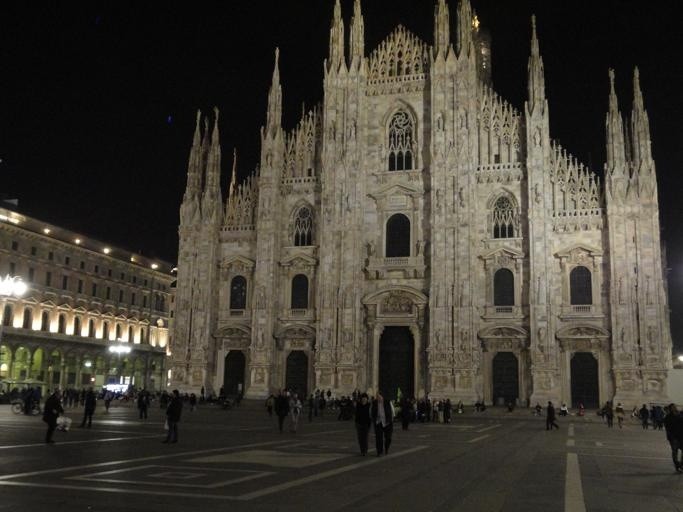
[56,416,72,431]
[164,419,169,430]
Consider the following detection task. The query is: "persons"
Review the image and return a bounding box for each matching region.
[349,391,370,459]
[364,391,397,456]
[42,389,64,445]
[165,387,180,442]
[660,400,681,477]
[1,381,682,432]
